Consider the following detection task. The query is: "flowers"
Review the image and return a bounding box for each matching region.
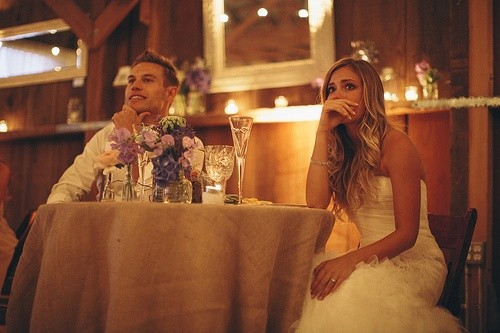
[416,60,439,82]
[94,114,191,180]
[178,58,211,92]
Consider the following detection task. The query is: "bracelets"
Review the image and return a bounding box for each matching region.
[309,159,329,166]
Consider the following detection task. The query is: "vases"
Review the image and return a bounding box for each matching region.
[163,168,193,203]
[423,83,438,99]
[152,176,163,202]
[100,172,118,201]
[121,165,139,202]
[170,95,186,115]
[189,93,207,113]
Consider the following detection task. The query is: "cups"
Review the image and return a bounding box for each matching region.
[67,96,82,124]
[205,145,237,187]
[201,174,226,205]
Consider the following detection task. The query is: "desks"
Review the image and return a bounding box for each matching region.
[38,204,336,333]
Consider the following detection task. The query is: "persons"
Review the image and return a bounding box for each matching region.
[46,48,204,204]
[294,58,464,333]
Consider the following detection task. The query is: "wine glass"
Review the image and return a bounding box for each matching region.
[133,122,150,203]
[228,116,254,205]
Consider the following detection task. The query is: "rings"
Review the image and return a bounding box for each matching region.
[330,278,336,283]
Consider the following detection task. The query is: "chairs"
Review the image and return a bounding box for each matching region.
[427,208,478,307]
